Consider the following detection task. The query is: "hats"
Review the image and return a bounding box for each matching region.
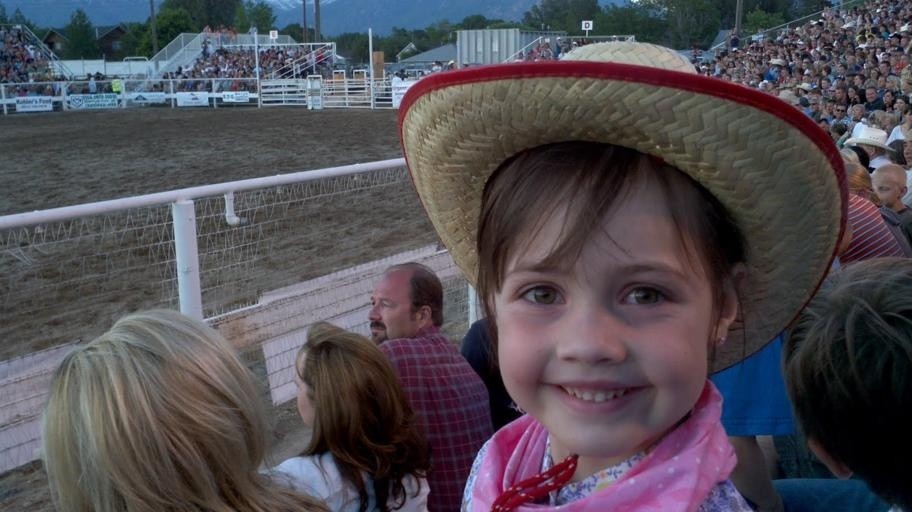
[841,121,898,156]
[731,2,909,113]
[391,38,850,381]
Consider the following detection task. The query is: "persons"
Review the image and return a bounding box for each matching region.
[453,141,756,510]
[150,28,333,92]
[0,28,122,97]
[271,322,440,510]
[458,313,520,432]
[383,33,634,86]
[31,305,335,512]
[692,0,912,510]
[362,259,492,508]
